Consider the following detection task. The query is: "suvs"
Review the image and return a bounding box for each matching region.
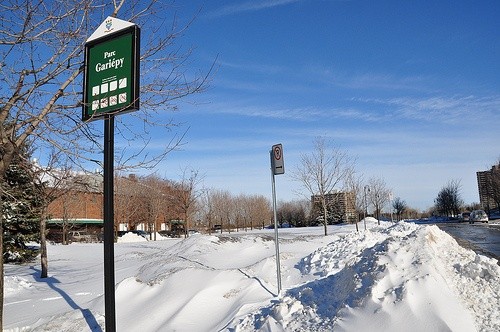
[67,231,91,242]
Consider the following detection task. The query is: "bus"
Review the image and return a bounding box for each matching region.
[45,219,106,243]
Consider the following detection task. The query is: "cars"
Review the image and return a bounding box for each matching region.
[468,209,489,224]
[461,211,471,222]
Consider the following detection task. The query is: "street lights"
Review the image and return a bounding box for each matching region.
[363,184,370,230]
